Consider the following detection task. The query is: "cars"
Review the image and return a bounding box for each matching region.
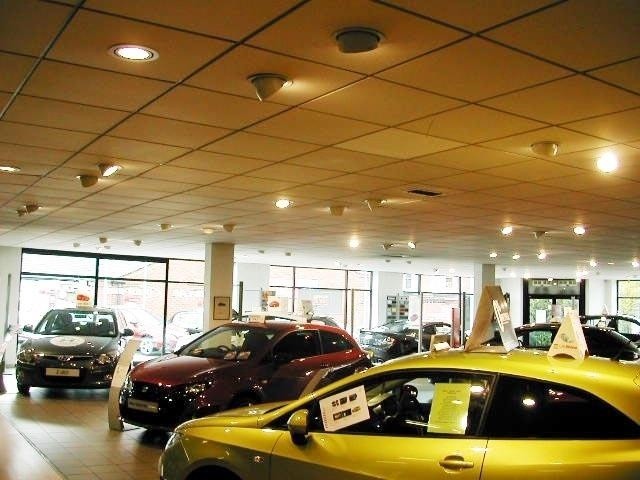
[113,306,178,355]
[6,305,137,396]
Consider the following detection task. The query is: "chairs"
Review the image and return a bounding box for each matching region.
[242,331,268,358]
[55,311,73,329]
[98,318,110,326]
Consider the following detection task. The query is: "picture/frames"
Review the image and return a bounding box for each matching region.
[212,296,231,321]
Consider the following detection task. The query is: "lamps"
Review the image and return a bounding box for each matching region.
[242,72,296,102]
[333,28,384,54]
